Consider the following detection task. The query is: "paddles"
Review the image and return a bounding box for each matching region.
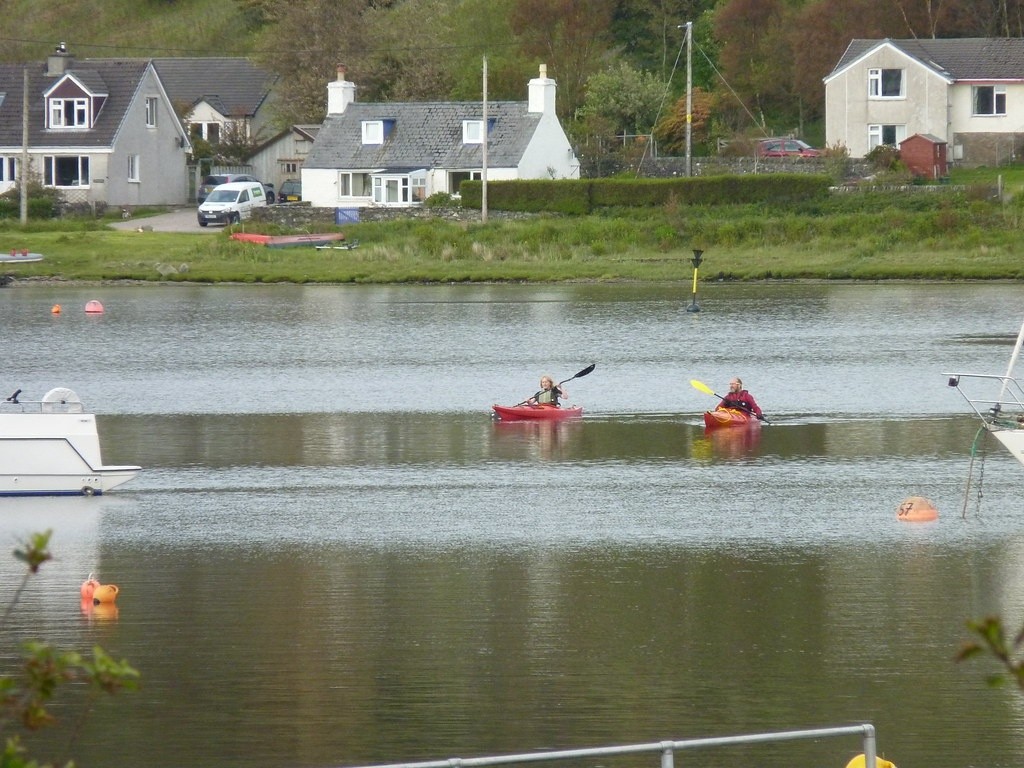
[511,364,595,407]
[690,379,772,425]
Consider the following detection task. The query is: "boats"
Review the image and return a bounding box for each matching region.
[703,412,761,430]
[228,232,344,251]
[493,405,584,421]
[940,371,1023,465]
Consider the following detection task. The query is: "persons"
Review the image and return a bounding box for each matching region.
[715,378,763,422]
[525,376,568,409]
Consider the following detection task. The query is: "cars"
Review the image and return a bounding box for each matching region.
[277,179,302,204]
[199,174,276,205]
[198,182,267,226]
[758,140,825,157]
